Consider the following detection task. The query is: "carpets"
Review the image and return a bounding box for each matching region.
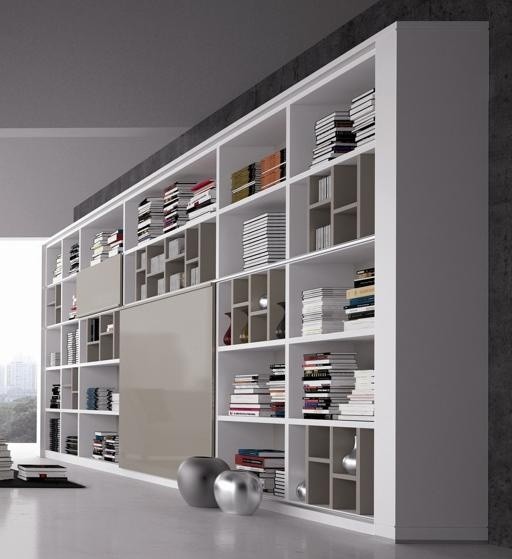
[0,469,87,488]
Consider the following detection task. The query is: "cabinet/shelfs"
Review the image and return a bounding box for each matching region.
[40,18,490,544]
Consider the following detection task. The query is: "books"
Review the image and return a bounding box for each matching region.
[344,268,374,332]
[318,175,331,200]
[229,364,285,418]
[0,437,71,487]
[136,176,214,300]
[316,225,331,251]
[241,212,286,272]
[235,449,285,496]
[302,288,350,335]
[46,227,124,463]
[308,88,375,170]
[301,352,375,422]
[229,148,286,203]
